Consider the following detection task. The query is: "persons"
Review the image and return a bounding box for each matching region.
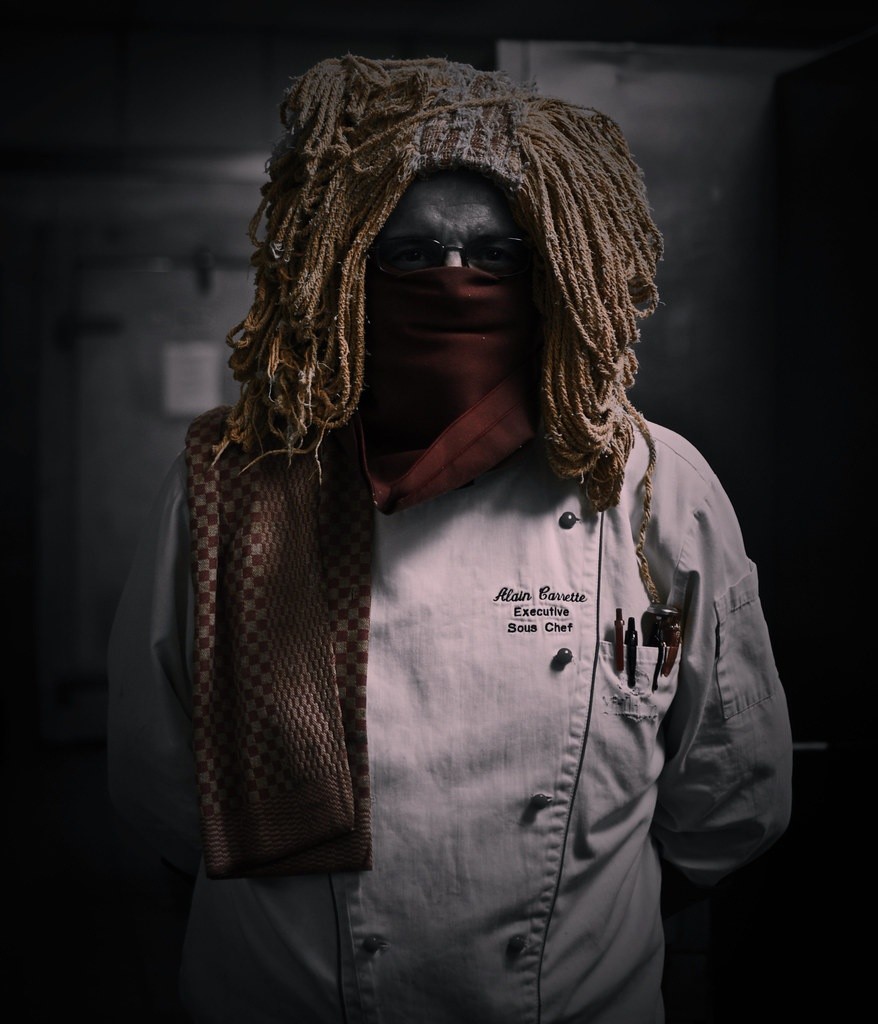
[111,52,797,1024]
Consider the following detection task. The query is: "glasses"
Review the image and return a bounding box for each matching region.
[365,233,534,279]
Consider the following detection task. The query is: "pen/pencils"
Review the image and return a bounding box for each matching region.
[606,607,687,692]
